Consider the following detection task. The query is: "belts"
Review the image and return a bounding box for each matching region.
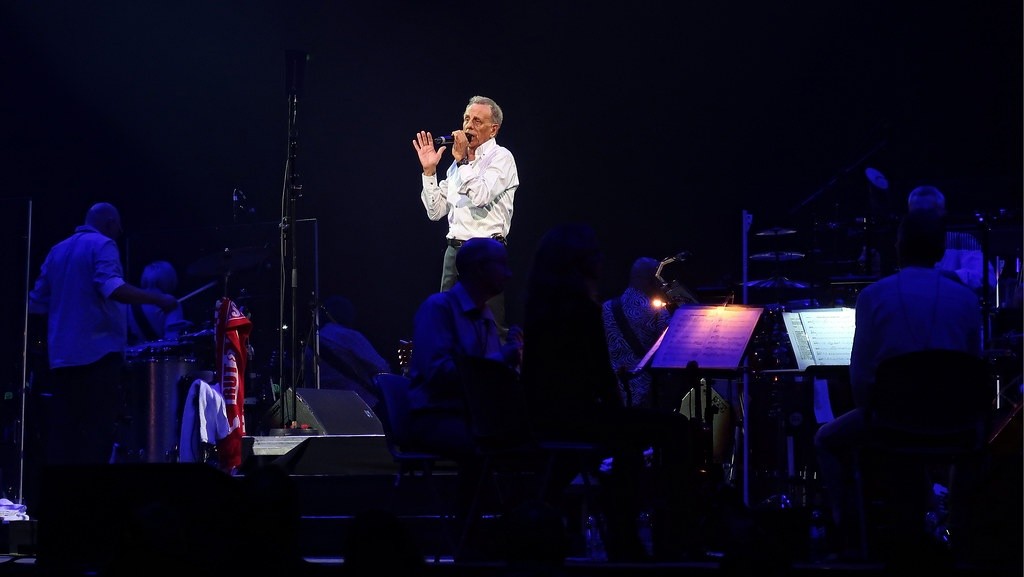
[447,237,506,247]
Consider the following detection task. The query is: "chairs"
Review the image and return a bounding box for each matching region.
[371,371,601,564]
[853,350,987,562]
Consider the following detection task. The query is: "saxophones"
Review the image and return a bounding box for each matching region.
[653,248,738,309]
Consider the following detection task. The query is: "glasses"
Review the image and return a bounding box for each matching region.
[117,222,124,239]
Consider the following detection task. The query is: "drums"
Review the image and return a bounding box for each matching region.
[180,327,250,374]
[121,356,198,458]
[123,338,196,359]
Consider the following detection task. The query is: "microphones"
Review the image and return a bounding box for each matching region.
[233,190,238,218]
[436,134,471,143]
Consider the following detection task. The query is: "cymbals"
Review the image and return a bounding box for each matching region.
[736,274,822,289]
[755,225,798,237]
[749,251,806,261]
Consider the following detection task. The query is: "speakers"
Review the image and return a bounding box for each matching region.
[260,386,384,435]
[36,461,301,577]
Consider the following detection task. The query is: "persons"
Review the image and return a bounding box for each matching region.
[909,186,997,298]
[32,460,1022,576]
[410,237,526,380]
[28,201,181,466]
[600,257,676,407]
[304,293,390,410]
[128,259,183,340]
[413,95,518,294]
[814,210,988,494]
